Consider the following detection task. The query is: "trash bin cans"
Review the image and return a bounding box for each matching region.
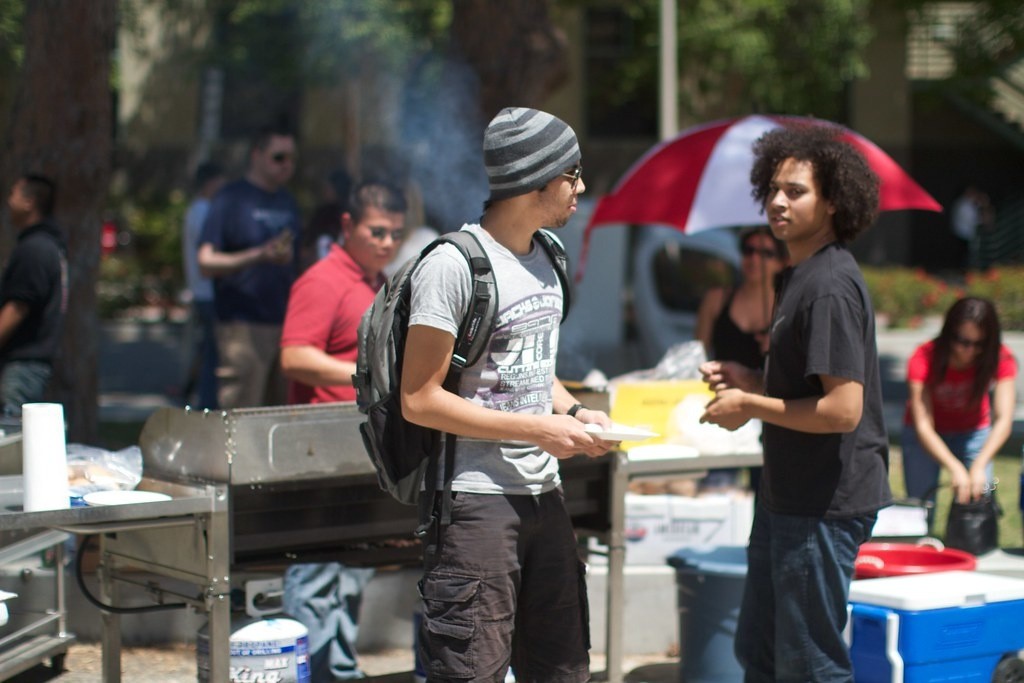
[668,545,749,683]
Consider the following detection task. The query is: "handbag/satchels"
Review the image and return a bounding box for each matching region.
[945,483,1001,556]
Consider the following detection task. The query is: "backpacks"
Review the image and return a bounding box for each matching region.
[356,230,570,507]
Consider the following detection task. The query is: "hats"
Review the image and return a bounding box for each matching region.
[484,107,582,200]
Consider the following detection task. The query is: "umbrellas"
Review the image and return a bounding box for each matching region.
[572,113,944,329]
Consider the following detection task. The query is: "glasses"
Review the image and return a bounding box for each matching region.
[956,337,984,349]
[742,245,776,259]
[559,166,582,187]
[264,150,296,164]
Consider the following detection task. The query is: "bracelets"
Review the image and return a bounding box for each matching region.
[565,403,590,418]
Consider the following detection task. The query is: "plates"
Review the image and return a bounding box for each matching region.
[83,490,172,507]
[584,423,658,443]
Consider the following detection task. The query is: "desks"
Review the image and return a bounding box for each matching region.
[608,441,809,683]
[0,455,231,683]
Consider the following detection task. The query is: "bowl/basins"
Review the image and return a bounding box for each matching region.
[0,474,23,506]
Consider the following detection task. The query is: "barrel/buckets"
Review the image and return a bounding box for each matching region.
[666,546,747,683]
[853,536,977,581]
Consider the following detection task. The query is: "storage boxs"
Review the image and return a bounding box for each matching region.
[582,493,755,655]
[844,570,1024,683]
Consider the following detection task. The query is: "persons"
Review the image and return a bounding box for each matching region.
[695,118,896,683]
[0,173,72,418]
[182,123,445,409]
[400,106,624,683]
[279,181,409,683]
[900,296,1019,537]
